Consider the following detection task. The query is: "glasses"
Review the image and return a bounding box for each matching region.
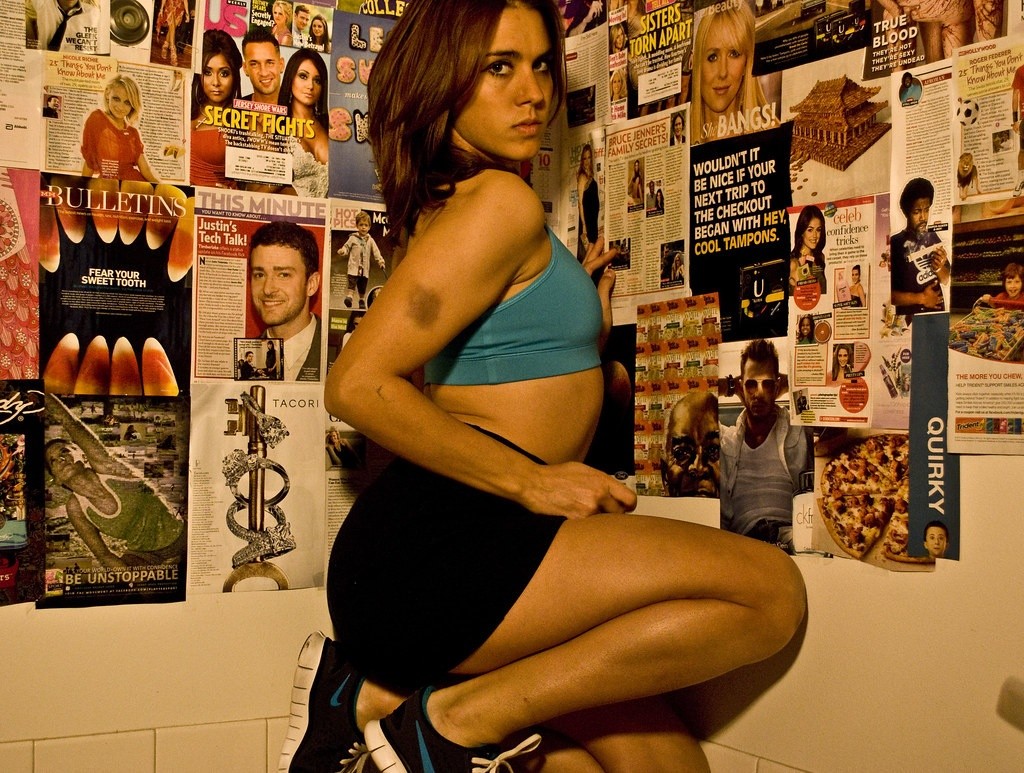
[742,378,775,395]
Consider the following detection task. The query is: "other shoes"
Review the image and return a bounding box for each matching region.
[359,299,365,309]
[344,297,352,308]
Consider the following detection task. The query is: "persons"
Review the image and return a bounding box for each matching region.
[981,263,1024,313]
[690,1,780,146]
[189,26,329,198]
[31,1,99,54]
[796,314,817,345]
[796,390,809,413]
[236,221,322,382]
[293,4,331,53]
[337,211,385,309]
[900,72,922,102]
[272,0,293,47]
[610,113,684,284]
[609,0,627,102]
[789,205,826,296]
[995,64,1024,198]
[660,339,833,558]
[341,311,366,350]
[890,177,951,316]
[576,145,604,289]
[278,0,807,773]
[45,393,185,582]
[158,0,190,67]
[923,521,950,559]
[80,74,161,183]
[850,265,866,308]
[833,344,853,381]
[558,0,607,38]
[43,96,59,118]
[326,426,361,468]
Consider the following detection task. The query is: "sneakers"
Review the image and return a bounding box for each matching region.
[276,630,369,773]
[364,684,540,773]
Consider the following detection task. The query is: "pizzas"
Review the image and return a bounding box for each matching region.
[816,434,937,563]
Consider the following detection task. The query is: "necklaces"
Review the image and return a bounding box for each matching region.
[196,103,230,128]
